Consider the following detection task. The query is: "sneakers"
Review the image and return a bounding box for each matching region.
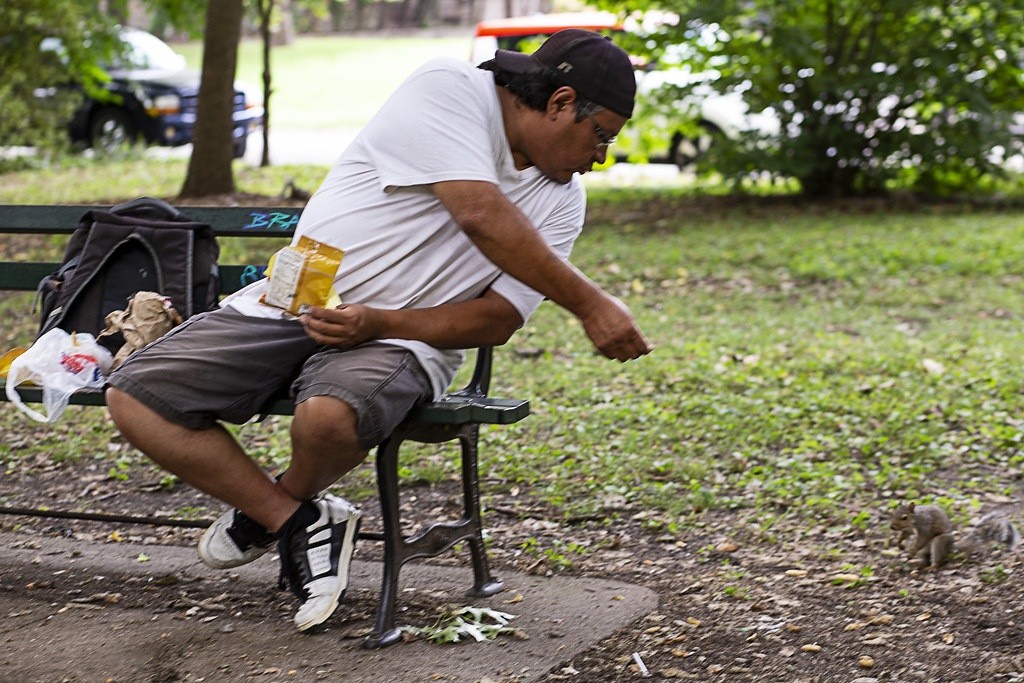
[197,465,331,570]
[278,492,365,633]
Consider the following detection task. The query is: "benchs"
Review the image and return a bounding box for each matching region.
[0,204,532,648]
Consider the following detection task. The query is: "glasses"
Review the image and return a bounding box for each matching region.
[578,95,618,151]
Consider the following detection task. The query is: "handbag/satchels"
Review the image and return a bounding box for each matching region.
[5,327,114,424]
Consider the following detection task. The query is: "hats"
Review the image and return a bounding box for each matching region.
[495,28,637,119]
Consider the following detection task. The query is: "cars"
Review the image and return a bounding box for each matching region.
[609,73,780,172]
[37,26,261,157]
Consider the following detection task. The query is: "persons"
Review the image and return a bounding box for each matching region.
[100,29,657,631]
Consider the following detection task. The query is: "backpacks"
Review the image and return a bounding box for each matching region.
[32,195,222,359]
